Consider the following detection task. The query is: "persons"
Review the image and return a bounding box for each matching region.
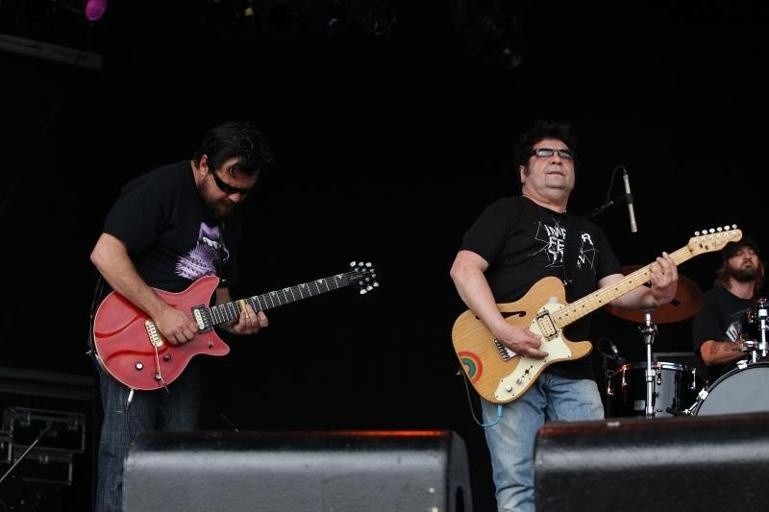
[90,118,270,512]
[450,120,680,512]
[691,236,769,395]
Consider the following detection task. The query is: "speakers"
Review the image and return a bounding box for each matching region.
[532,410,769,512]
[123,430,475,512]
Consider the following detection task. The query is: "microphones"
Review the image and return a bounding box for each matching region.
[619,170,640,234]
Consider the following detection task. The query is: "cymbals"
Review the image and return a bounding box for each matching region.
[602,265,706,324]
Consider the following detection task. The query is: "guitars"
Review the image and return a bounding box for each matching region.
[451,225,742,406]
[93,261,378,392]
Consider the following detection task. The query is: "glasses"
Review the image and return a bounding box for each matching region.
[212,173,254,196]
[528,146,575,161]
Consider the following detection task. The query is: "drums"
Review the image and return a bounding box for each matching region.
[689,359,769,417]
[610,362,695,419]
[674,297,768,417]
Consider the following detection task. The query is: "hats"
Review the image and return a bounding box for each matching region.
[724,237,760,257]
